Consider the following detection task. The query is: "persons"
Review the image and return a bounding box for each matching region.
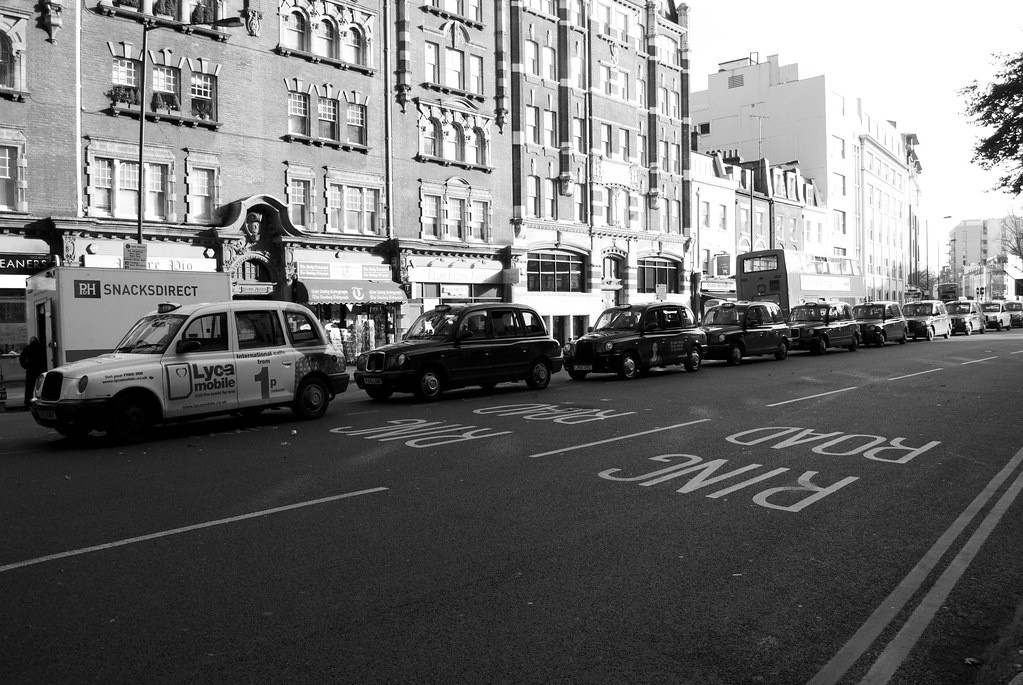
[17,337,47,412]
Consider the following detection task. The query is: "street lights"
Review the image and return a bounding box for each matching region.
[135,16,243,246]
[937,238,957,300]
[924,215,953,300]
[749,160,800,253]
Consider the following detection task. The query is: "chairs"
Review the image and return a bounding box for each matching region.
[724,313,737,323]
[644,313,659,330]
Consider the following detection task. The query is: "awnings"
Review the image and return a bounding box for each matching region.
[291,278,408,306]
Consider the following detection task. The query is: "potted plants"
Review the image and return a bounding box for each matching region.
[195,104,212,120]
[169,95,182,116]
[129,89,141,110]
[154,92,168,114]
[109,87,129,109]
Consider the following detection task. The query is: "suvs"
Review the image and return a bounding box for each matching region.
[980,300,1012,331]
[27,299,350,443]
[563,302,709,381]
[945,300,987,336]
[1005,301,1023,328]
[697,301,792,365]
[901,300,953,342]
[784,301,863,354]
[851,300,909,347]
[353,302,565,403]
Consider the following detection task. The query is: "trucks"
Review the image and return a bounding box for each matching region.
[24,253,274,392]
